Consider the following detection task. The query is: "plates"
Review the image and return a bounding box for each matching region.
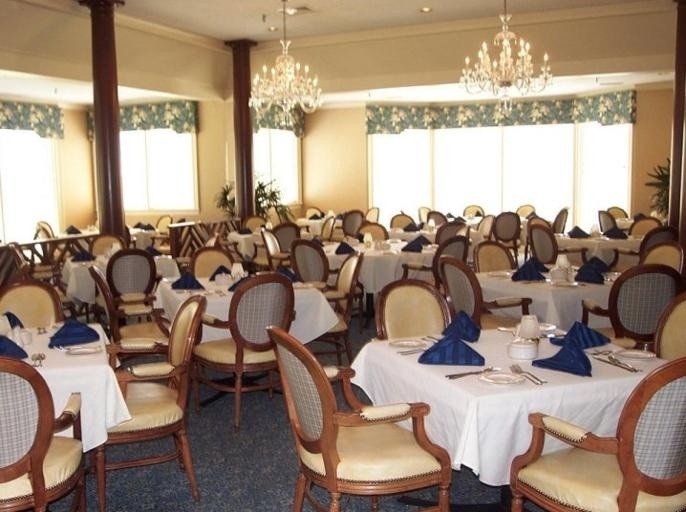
[615,349,657,361]
[517,322,557,332]
[64,347,97,355]
[480,367,527,383]
[489,270,510,278]
[388,339,426,349]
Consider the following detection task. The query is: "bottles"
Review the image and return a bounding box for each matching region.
[362,232,401,251]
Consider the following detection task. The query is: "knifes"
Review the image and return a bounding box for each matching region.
[591,357,634,372]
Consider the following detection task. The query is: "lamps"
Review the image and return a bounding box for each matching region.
[457,0,554,113]
[246,0,325,118]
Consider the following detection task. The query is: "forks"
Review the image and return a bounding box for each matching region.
[509,364,549,386]
[444,366,495,380]
[513,363,548,383]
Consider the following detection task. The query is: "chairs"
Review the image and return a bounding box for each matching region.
[507,356,685,512]
[264,323,454,512]
[0,205,686,367]
[91,293,208,512]
[192,271,297,429]
[0,354,92,512]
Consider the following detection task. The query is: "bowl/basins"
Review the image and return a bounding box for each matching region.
[550,254,578,287]
[509,337,538,360]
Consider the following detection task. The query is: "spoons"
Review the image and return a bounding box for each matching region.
[31,352,46,369]
[608,353,640,372]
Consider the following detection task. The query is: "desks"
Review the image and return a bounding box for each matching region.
[1,314,129,510]
[348,322,669,511]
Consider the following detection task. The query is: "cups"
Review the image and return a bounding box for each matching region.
[0,314,32,350]
[213,272,248,285]
[602,272,622,281]
[521,315,539,335]
